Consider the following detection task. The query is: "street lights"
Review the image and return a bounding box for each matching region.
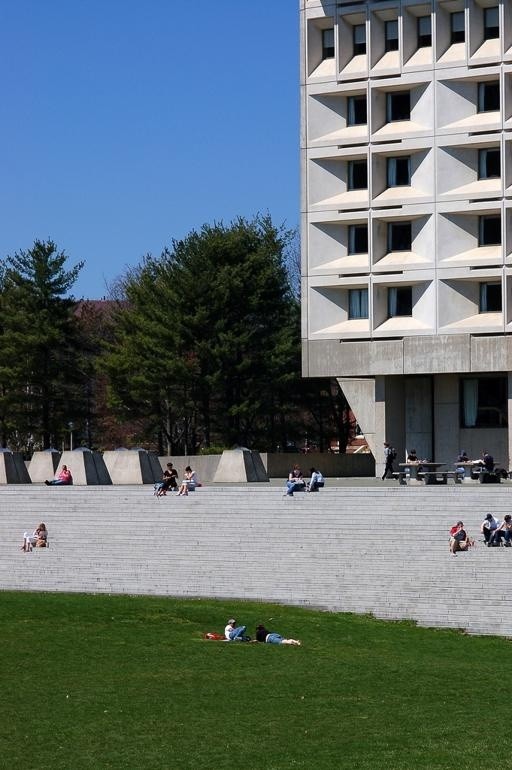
[67,420,75,450]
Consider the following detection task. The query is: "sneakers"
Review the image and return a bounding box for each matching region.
[156,491,166,496]
[503,541,511,547]
[176,491,188,496]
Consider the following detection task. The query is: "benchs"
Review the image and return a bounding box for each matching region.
[394,461,512,485]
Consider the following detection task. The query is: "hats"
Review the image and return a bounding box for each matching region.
[485,514,492,520]
[228,620,235,624]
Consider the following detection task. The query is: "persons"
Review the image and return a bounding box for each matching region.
[44,464,71,486]
[286,464,323,496]
[155,463,199,496]
[480,513,512,547]
[380,442,398,481]
[407,449,425,481]
[224,619,251,642]
[249,625,301,646]
[475,449,494,484]
[20,522,48,553]
[455,449,469,480]
[448,521,471,557]
